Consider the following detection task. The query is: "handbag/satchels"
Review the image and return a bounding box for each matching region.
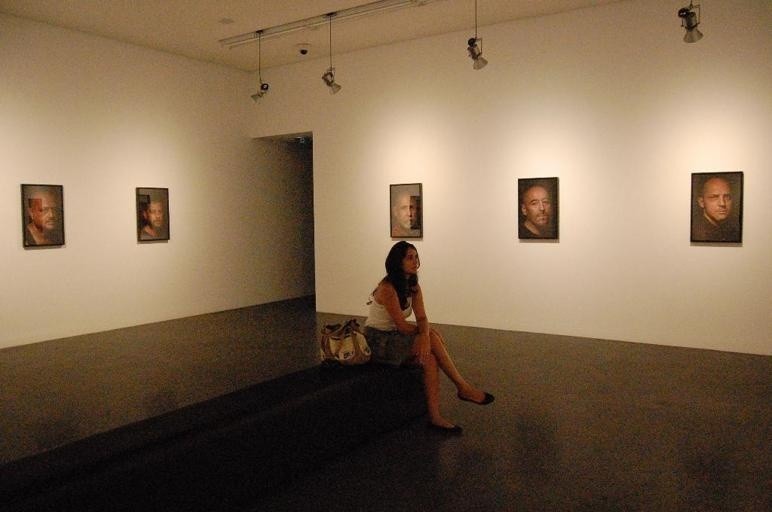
[319,318,371,365]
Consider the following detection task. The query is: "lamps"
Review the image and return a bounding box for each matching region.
[217,0,704,105]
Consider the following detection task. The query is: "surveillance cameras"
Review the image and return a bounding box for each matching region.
[297,42,312,55]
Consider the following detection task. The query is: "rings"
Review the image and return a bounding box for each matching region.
[426,351,430,355]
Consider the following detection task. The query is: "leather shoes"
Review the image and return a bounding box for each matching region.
[457,390,494,404]
[427,419,462,433]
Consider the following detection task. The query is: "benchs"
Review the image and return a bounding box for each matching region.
[0,362,426,512]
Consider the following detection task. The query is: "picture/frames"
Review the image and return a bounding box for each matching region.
[390,183,422,238]
[20,184,65,247]
[690,171,743,243]
[136,187,170,241]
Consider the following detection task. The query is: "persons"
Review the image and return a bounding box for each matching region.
[392,190,421,236]
[140,193,169,240]
[363,241,495,437]
[692,177,735,240]
[24,187,63,245]
[520,185,553,237]
